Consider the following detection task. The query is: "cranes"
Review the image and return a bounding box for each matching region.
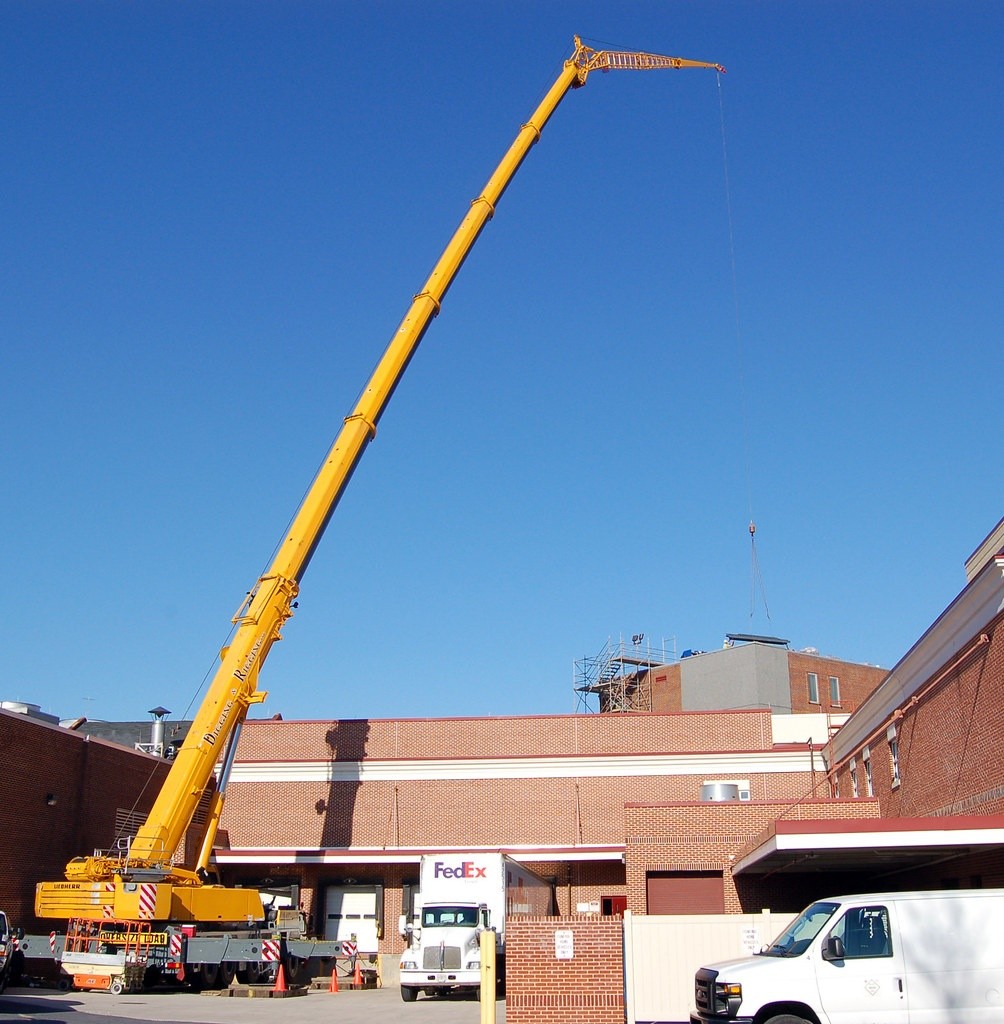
[32,32,793,988]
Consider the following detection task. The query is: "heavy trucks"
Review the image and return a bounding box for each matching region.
[397,851,553,1001]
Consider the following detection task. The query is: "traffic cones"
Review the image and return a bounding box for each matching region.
[329,969,338,992]
[272,964,285,992]
[353,966,363,985]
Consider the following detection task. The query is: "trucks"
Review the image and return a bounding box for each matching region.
[0,911,14,992]
[689,889,1004,1024]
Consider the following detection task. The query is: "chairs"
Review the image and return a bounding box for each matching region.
[853,916,888,955]
